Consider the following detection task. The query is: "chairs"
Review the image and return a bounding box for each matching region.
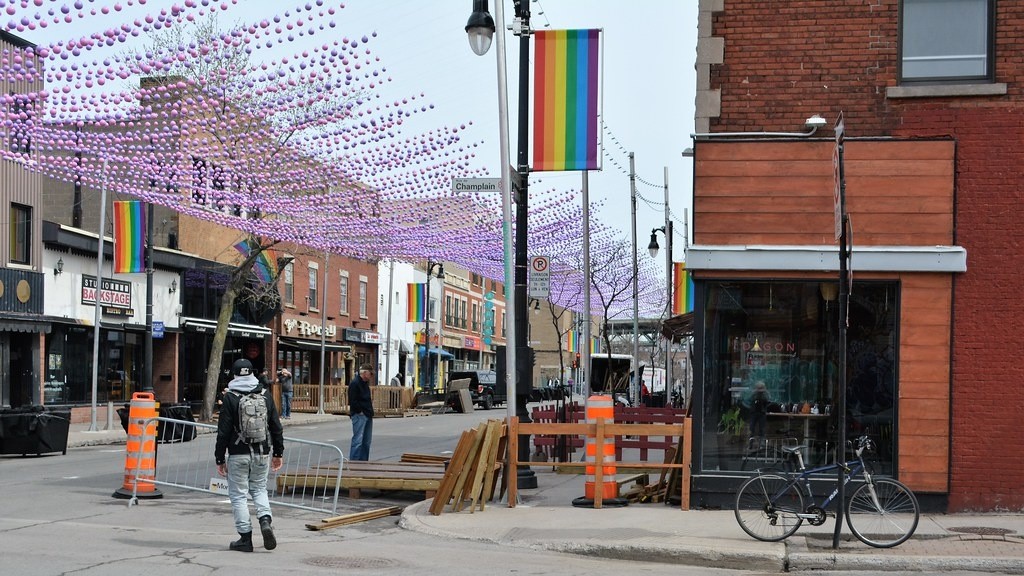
[109,380,122,399]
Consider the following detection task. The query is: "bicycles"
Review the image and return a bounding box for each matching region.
[734,435,920,548]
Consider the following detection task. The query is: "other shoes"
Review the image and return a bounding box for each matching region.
[285,416,290,419]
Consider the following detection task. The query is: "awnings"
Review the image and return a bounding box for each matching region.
[281,337,351,352]
[419,345,455,361]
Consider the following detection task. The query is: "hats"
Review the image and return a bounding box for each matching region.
[232,358,253,376]
[757,383,764,392]
[360,363,374,371]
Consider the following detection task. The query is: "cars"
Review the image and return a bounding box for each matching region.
[446,370,496,413]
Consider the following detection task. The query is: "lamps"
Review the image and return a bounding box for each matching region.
[54,257,63,275]
[327,316,335,320]
[352,320,360,323]
[370,324,377,326]
[300,312,309,316]
[168,279,177,293]
[804,115,828,128]
[681,148,693,157]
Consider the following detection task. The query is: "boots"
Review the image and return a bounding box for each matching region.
[230,531,253,552]
[259,515,276,550]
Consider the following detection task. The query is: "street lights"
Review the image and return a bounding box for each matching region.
[465,0,538,489]
[424,263,446,390]
[648,227,672,400]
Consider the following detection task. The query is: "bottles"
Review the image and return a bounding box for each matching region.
[813,404,819,414]
[793,404,800,414]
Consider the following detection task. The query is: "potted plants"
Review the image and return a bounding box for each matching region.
[717,407,747,472]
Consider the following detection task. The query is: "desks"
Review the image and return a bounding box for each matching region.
[766,412,831,465]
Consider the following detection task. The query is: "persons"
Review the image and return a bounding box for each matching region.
[390,373,402,409]
[642,380,652,403]
[546,377,573,392]
[65,363,125,402]
[748,380,771,450]
[214,359,285,553]
[258,368,293,420]
[348,363,376,459]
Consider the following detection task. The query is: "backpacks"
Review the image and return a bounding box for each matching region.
[228,388,267,444]
[752,392,766,410]
